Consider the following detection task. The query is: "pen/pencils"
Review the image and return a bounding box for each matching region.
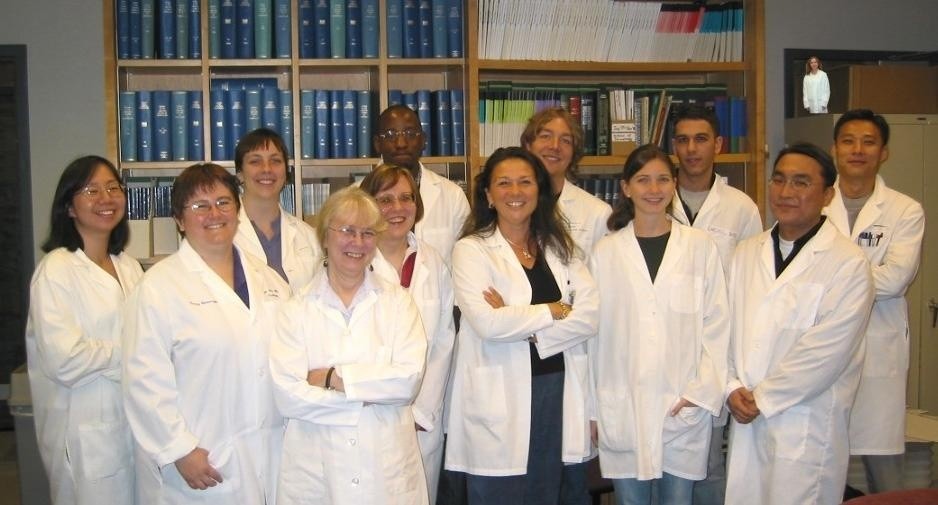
[860,231,883,247]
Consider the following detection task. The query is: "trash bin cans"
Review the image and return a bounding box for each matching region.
[8,364,52,505]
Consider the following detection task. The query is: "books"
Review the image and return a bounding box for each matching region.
[298,1,381,215]
[117,1,205,220]
[387,1,468,195]
[208,1,296,217]
[478,79,747,206]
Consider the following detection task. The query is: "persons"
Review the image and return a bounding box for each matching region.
[27,101,928,505]
[802,55,831,114]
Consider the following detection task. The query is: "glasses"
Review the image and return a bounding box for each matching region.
[181,197,239,214]
[766,174,825,193]
[72,180,128,201]
[374,192,417,210]
[378,128,421,141]
[326,224,376,244]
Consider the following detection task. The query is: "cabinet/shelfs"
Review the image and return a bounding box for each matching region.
[102,0,469,273]
[785,114,936,444]
[468,0,770,231]
